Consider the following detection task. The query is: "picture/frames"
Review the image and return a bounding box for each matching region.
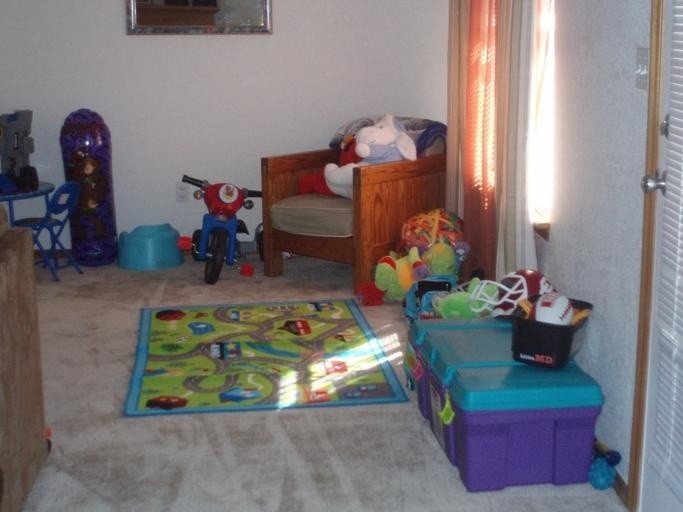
[125,0,273,35]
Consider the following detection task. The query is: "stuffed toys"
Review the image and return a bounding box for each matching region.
[322,110,418,200]
[295,133,364,197]
[403,273,460,319]
[433,276,500,320]
[353,241,473,308]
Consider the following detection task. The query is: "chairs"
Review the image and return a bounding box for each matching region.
[14,179,84,282]
[261,116,447,296]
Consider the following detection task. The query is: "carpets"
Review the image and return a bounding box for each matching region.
[120,296,411,417]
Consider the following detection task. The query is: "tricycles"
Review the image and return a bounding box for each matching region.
[177,172,269,286]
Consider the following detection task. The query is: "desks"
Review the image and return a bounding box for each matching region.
[0,175,58,266]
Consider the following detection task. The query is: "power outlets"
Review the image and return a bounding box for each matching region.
[174,180,189,204]
